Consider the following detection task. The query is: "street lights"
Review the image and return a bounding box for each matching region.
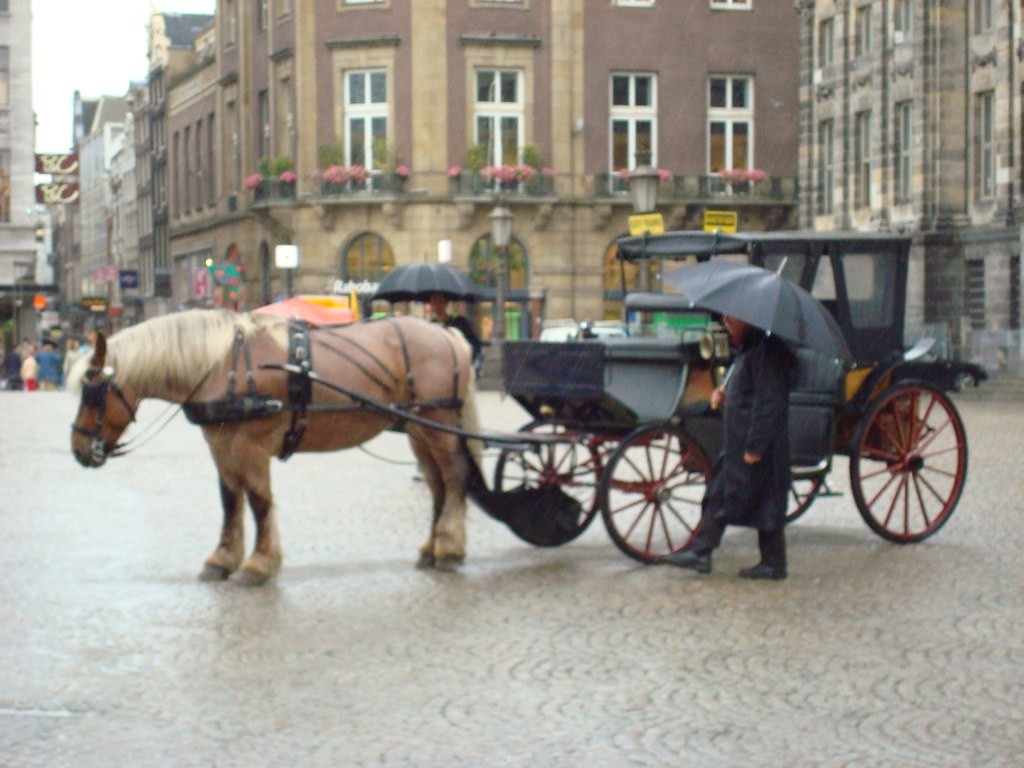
[479,196,513,390]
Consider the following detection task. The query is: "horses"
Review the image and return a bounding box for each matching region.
[64,310,482,587]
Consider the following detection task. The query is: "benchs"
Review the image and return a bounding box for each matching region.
[787,350,839,456]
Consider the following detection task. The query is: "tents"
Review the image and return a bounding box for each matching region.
[251,296,354,326]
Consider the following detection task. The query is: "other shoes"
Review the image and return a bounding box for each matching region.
[412,472,426,482]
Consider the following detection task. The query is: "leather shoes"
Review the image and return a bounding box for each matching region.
[664,549,712,573]
[738,555,788,580]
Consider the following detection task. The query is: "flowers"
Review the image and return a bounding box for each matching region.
[242,169,767,193]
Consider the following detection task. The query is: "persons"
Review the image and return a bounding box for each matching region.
[656,312,792,581]
[6,337,85,392]
[427,295,484,373]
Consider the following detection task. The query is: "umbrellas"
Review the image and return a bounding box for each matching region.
[657,253,853,410]
[370,253,483,306]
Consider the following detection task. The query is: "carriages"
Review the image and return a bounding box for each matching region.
[66,230,988,586]
